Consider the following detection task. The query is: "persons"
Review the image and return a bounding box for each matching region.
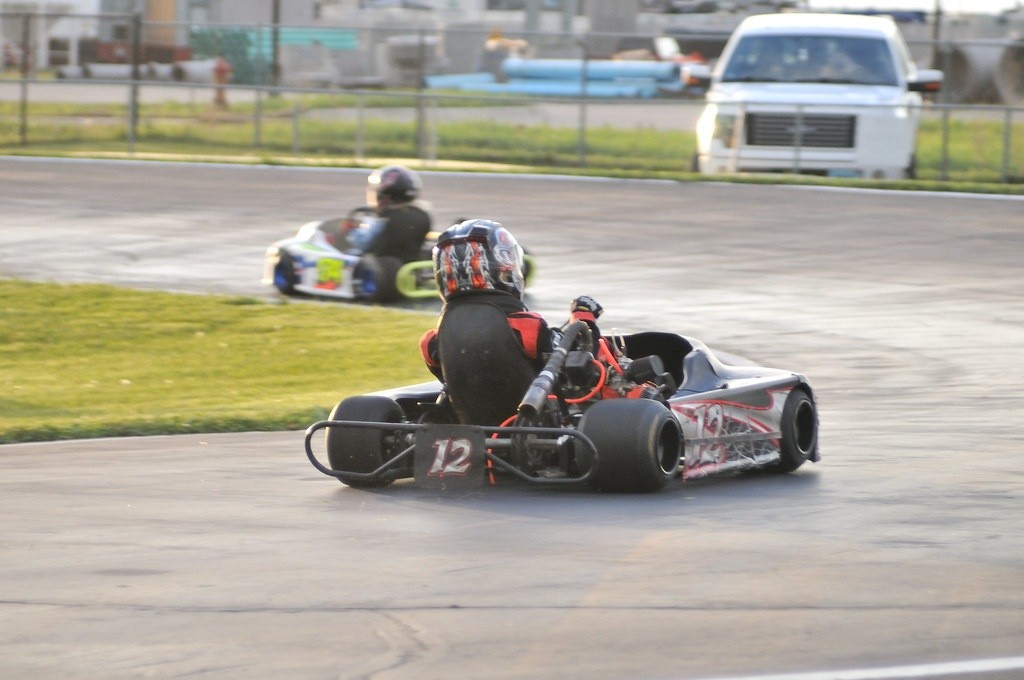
[420,218,661,397]
[333,168,428,249]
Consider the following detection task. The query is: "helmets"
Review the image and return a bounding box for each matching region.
[432,219,523,300]
[368,165,423,206]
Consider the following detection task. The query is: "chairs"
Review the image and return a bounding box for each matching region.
[356,203,431,264]
[437,299,560,440]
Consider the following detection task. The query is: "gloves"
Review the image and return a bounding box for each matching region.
[570,296,604,320]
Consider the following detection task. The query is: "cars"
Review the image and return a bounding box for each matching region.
[681,13,945,179]
